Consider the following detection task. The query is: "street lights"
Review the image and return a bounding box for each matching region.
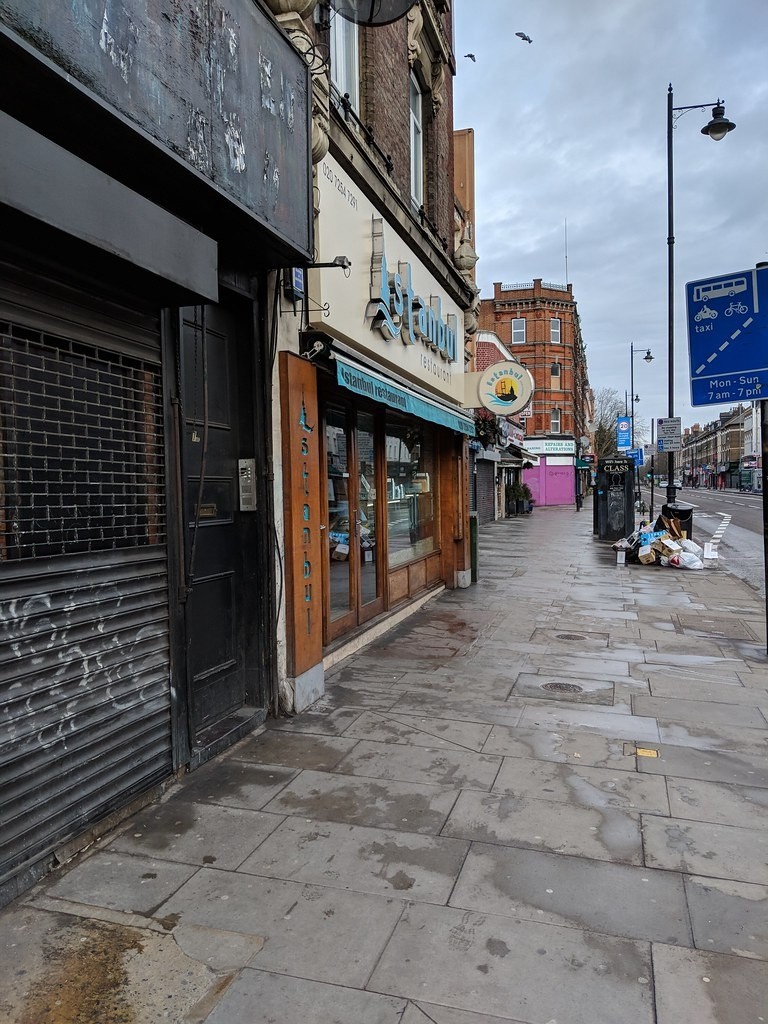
[624,390,643,418]
[662,83,739,543]
[630,342,655,492]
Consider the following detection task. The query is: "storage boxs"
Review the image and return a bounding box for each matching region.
[661,539,683,558]
[638,545,655,565]
[332,544,349,561]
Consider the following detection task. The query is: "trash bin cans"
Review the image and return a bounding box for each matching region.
[662,502,693,541]
[635,492,642,502]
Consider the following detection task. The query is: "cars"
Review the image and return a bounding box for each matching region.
[673,479,683,490]
[659,481,670,488]
